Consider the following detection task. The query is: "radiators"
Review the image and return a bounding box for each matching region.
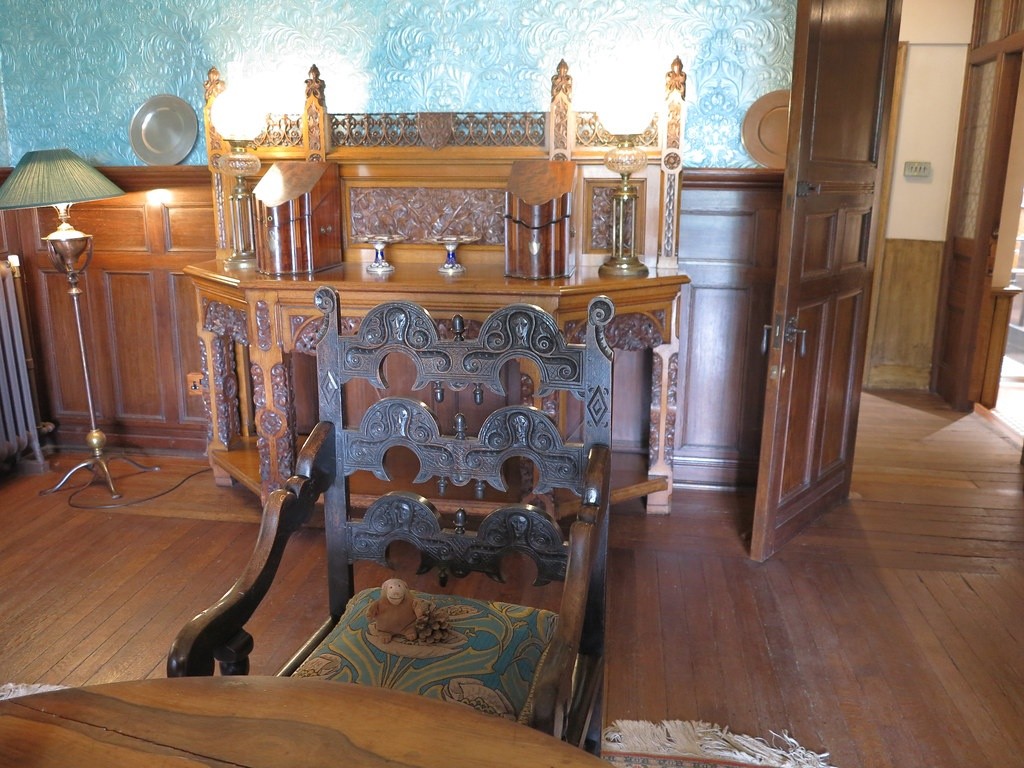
[0,254,55,478]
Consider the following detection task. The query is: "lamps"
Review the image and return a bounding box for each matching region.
[210,85,265,272]
[597,79,655,277]
[0,148,161,499]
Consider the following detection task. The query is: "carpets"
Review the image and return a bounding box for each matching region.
[602,718,828,768]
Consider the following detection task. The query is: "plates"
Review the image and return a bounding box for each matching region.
[129,93,199,166]
[741,88,791,169]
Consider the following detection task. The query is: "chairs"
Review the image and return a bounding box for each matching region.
[167,286,616,757]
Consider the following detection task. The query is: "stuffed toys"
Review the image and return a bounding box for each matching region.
[366,580,451,644]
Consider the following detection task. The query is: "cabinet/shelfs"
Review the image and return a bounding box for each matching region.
[183,54,691,528]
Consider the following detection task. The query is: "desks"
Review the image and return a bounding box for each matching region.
[0,676,615,768]
